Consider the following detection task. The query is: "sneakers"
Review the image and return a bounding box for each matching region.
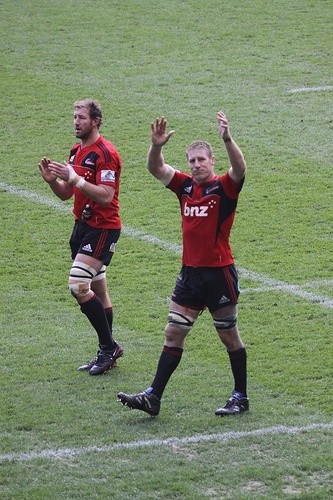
[89,341,123,375]
[215,390,249,414]
[77,356,98,371]
[117,387,160,416]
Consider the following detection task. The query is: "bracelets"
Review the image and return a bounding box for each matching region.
[224,136,231,142]
[76,178,86,189]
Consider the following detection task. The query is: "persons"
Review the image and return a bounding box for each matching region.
[116,111,249,416]
[38,98,123,376]
